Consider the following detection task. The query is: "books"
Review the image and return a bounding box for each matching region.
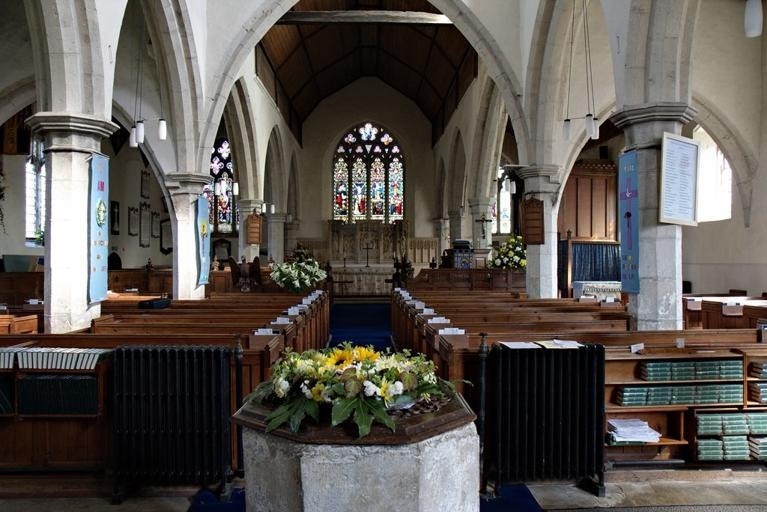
[606,418,662,446]
[617,384,744,406]
[640,361,744,381]
[0,348,115,369]
[751,360,767,403]
[696,413,767,460]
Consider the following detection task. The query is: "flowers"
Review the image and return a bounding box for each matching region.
[240,344,476,440]
[269,257,327,294]
[491,236,526,273]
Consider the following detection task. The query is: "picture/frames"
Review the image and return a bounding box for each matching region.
[658,131,700,227]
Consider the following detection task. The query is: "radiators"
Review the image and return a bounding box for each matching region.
[473,332,608,499]
[109,343,234,503]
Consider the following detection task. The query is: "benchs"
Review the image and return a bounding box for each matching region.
[390,286,766,465]
[1,291,334,496]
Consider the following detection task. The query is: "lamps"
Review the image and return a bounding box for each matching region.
[130,27,166,148]
